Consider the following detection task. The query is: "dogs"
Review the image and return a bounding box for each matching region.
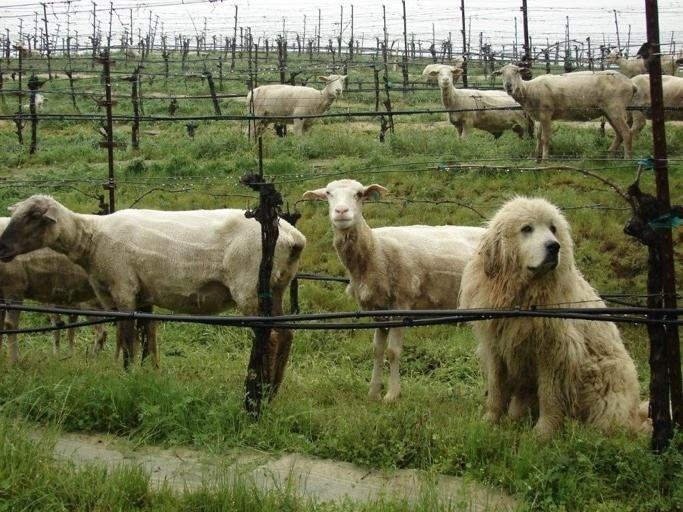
[459,194,653,440]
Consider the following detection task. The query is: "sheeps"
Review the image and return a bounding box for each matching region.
[23,93,48,122]
[422,43,683,165]
[303,178,490,404]
[0,193,304,406]
[245,74,348,137]
